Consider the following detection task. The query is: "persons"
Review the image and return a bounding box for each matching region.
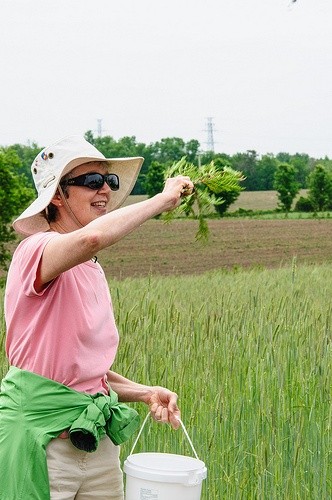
[4,134,193,500]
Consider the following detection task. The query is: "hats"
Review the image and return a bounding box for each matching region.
[12,135,145,236]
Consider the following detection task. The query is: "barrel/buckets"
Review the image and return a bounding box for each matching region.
[123,410,207,500]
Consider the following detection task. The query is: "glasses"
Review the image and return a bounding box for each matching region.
[61,172,120,191]
